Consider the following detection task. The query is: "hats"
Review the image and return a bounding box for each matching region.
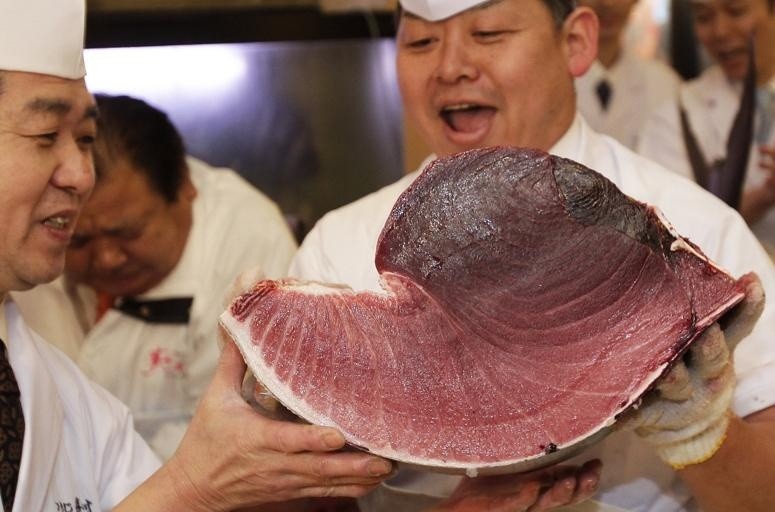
[401,1,501,23]
[1,2,88,79]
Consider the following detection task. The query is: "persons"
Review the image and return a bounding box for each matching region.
[242,0,775,512]
[635,0,775,260]
[11,91,299,462]
[573,0,688,160]
[0,54,605,512]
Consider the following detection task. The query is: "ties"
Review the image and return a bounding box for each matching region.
[594,78,615,113]
[0,341,25,512]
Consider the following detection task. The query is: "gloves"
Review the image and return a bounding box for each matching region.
[622,271,765,472]
